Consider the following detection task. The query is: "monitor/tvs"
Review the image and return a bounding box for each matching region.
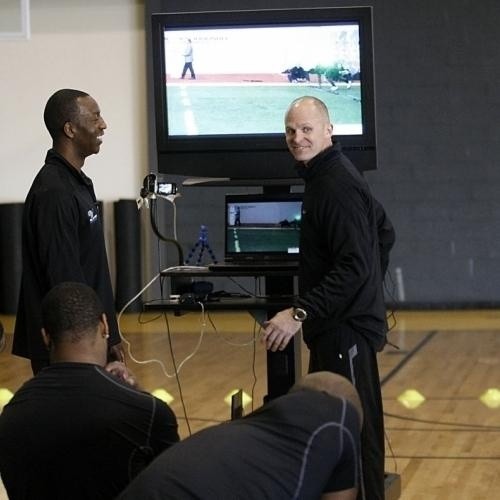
[151,5,378,187]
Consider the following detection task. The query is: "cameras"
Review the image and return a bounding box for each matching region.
[140,175,177,198]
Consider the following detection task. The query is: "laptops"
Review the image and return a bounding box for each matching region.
[208,192,304,271]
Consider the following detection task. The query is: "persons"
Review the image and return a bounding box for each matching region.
[10,88,124,377]
[260,95,396,500]
[0,282,182,500]
[180,37,197,79]
[233,206,243,226]
[117,370,364,500]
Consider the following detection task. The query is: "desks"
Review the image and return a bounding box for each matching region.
[143,265,300,398]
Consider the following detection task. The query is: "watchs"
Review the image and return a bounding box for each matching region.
[290,305,310,323]
